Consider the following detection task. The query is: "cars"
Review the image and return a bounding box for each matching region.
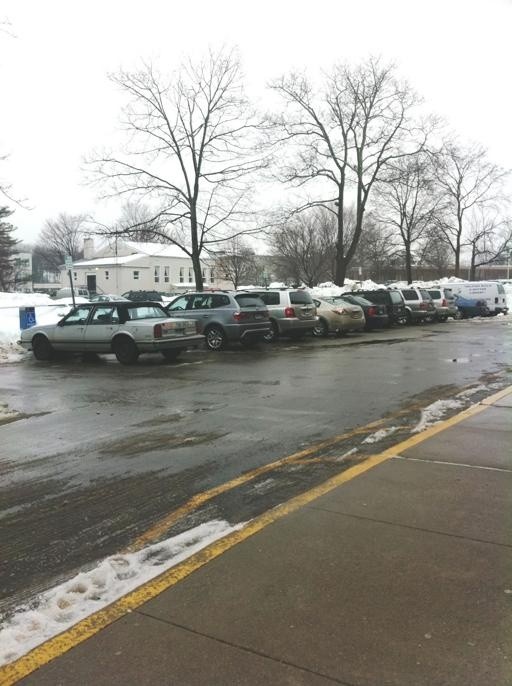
[19,300,206,364]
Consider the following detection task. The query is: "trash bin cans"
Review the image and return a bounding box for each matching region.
[19,307,36,328]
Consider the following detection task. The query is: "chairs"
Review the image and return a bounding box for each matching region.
[194,300,204,308]
[97,315,110,324]
